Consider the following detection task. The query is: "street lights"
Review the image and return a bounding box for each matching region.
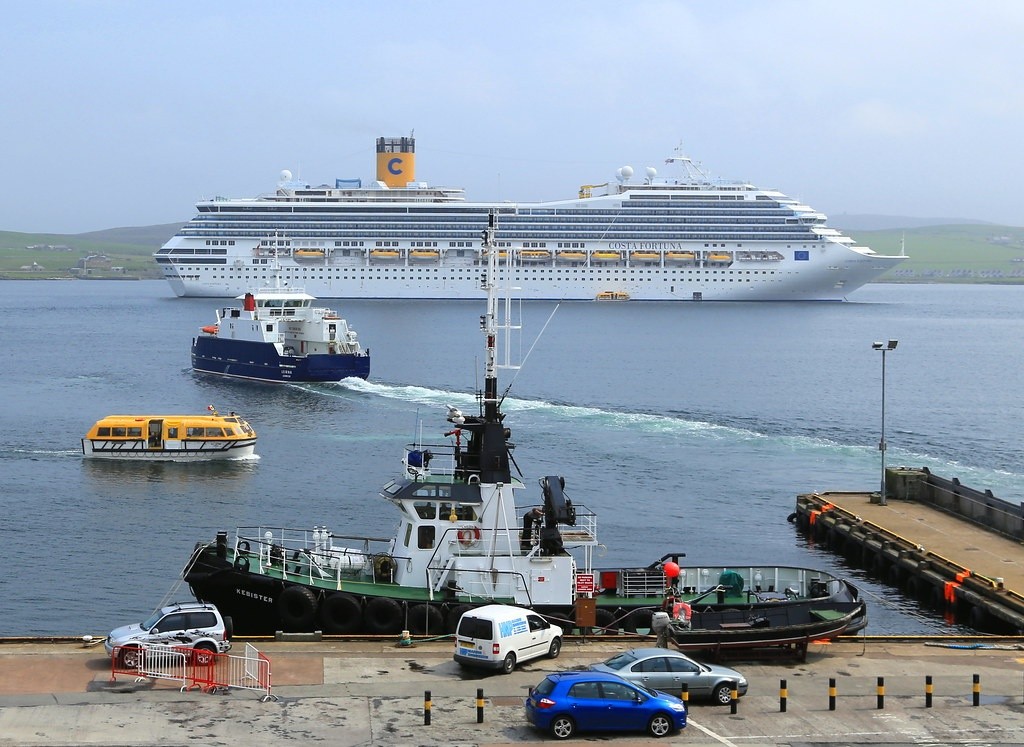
[871,339,899,506]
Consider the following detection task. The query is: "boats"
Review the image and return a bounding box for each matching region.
[370,251,399,260]
[707,255,732,264]
[556,252,586,263]
[629,253,661,263]
[484,252,508,263]
[409,252,439,260]
[81,404,257,458]
[294,251,324,260]
[521,251,552,263]
[666,252,695,262]
[181,205,870,637]
[191,228,372,385]
[590,252,620,263]
[595,290,631,303]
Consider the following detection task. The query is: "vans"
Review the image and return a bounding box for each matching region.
[453,605,564,676]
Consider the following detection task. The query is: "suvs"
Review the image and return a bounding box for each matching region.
[526,671,686,739]
[105,598,233,670]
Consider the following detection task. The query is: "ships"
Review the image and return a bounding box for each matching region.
[151,130,910,301]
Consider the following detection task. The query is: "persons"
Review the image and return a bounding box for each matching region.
[522,508,544,546]
[230,412,236,416]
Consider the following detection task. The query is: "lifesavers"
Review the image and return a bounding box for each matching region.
[673,604,692,620]
[459,523,480,547]
[319,593,363,634]
[449,605,473,635]
[278,585,317,630]
[405,604,445,639]
[625,610,658,637]
[581,609,619,637]
[547,612,573,636]
[366,597,406,634]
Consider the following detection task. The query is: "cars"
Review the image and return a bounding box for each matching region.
[589,647,749,706]
